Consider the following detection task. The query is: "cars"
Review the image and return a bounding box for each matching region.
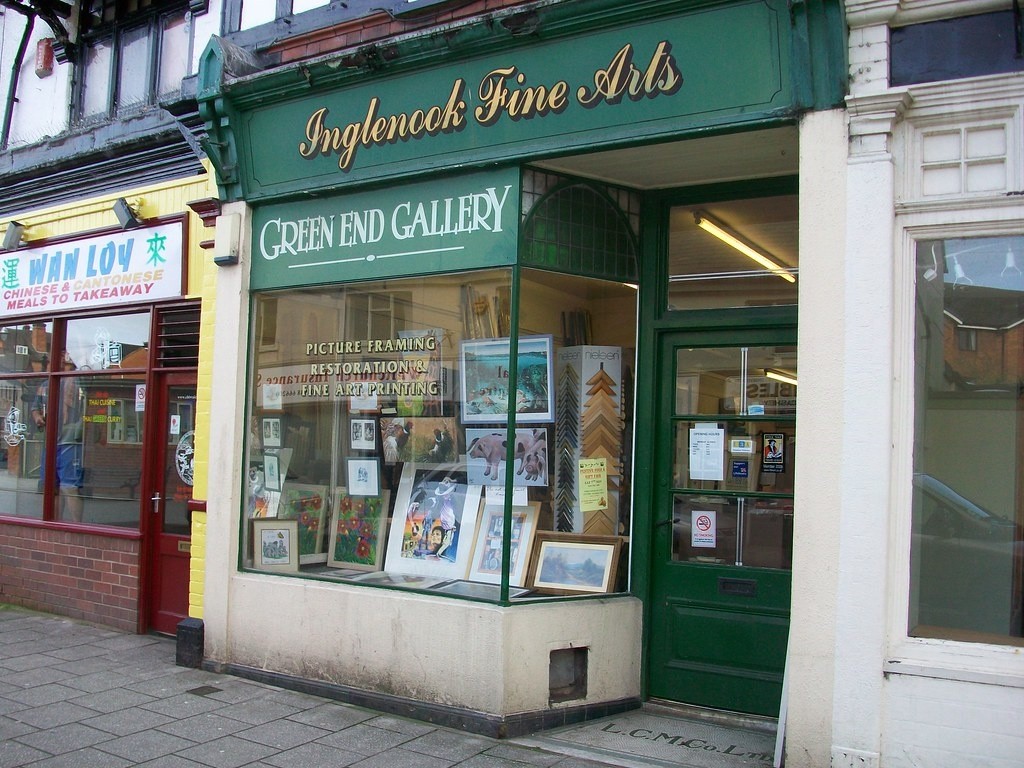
[907,473,1024,637]
[673,493,742,561]
[404,462,546,540]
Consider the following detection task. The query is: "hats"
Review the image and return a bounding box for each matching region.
[392,417,409,436]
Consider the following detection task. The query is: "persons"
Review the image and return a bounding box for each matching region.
[30,349,88,524]
